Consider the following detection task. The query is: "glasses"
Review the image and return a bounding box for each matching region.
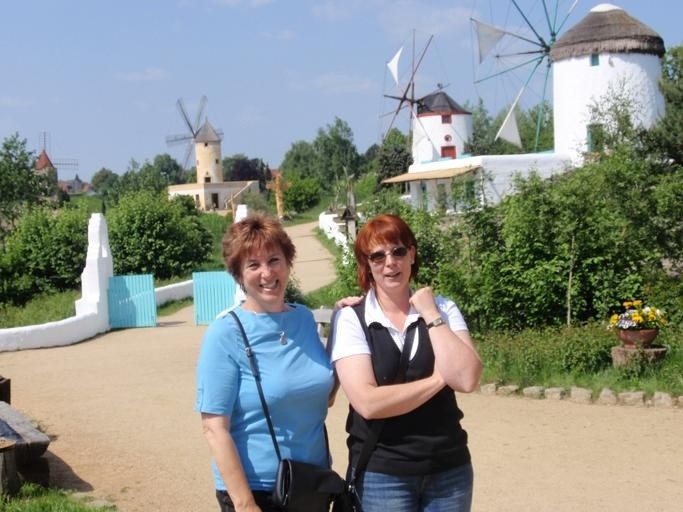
[367,246,408,263]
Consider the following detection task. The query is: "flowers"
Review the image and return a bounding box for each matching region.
[604,300,670,331]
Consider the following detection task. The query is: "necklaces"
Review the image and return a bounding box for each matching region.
[250,305,288,345]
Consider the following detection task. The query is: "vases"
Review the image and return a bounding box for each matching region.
[614,327,659,349]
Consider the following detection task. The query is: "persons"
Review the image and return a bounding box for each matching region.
[196,213,364,512]
[330,214,482,512]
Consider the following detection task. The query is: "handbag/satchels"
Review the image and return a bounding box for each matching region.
[272,458,362,512]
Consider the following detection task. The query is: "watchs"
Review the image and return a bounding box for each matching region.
[426,317,446,329]
